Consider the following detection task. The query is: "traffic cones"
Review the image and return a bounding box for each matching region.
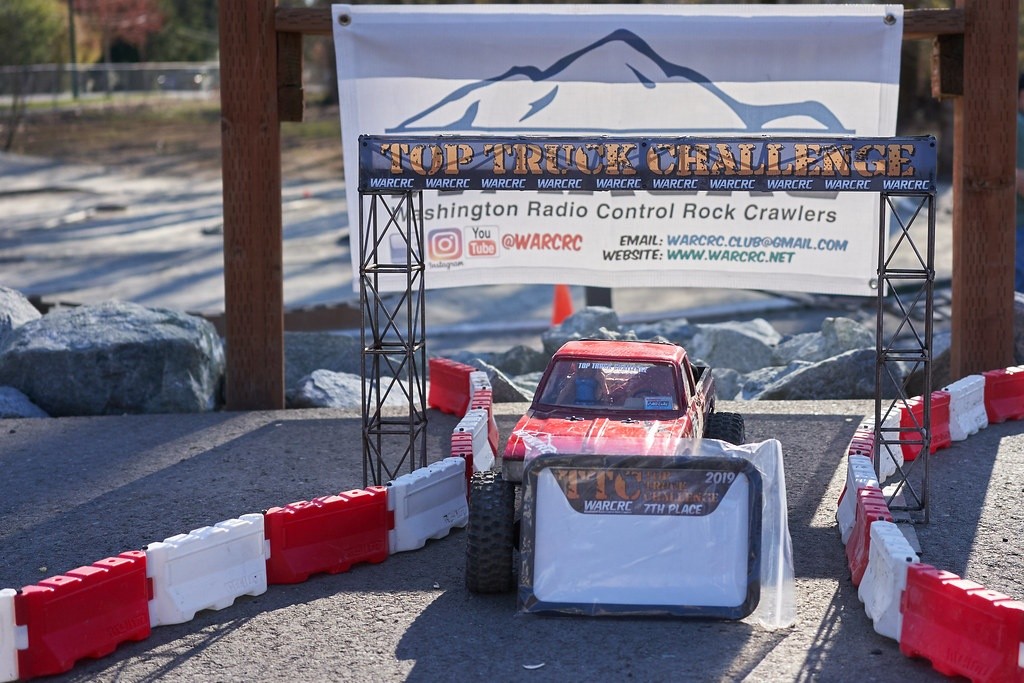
[549,280,576,331]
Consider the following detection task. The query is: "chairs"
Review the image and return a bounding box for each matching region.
[562,368,613,406]
[624,367,677,402]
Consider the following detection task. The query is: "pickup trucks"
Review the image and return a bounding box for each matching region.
[464,338,746,595]
